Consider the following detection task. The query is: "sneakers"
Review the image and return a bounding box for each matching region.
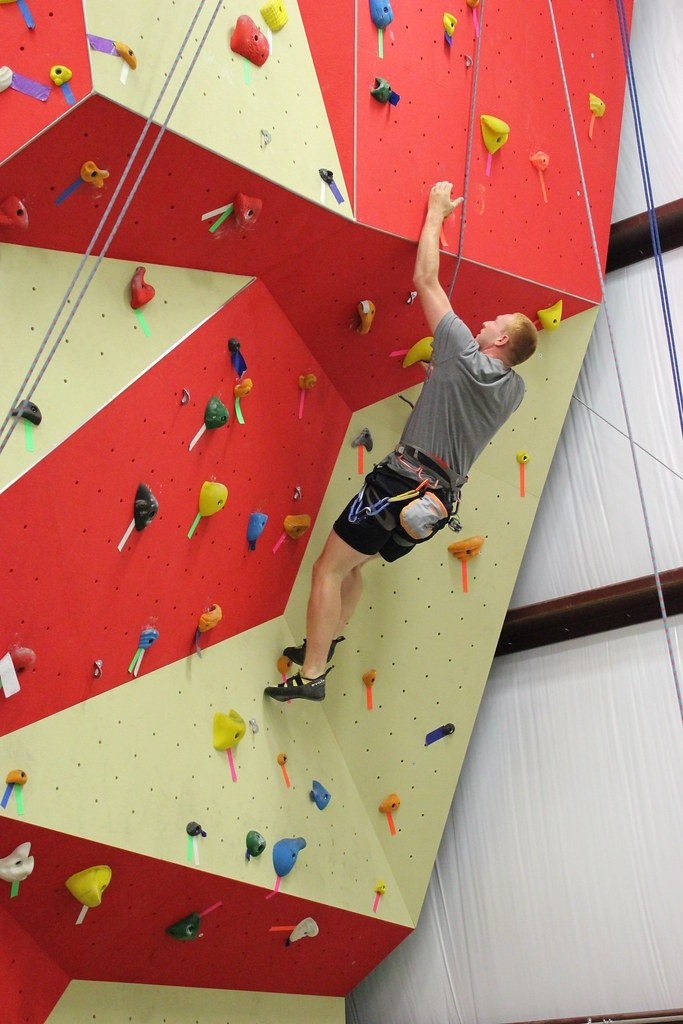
[265,666,335,702]
[283,636,345,666]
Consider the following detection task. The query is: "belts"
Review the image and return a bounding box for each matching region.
[405,445,463,489]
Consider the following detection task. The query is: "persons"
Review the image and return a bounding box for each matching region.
[263,181,538,702]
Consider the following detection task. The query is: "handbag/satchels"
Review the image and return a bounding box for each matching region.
[399,492,448,540]
